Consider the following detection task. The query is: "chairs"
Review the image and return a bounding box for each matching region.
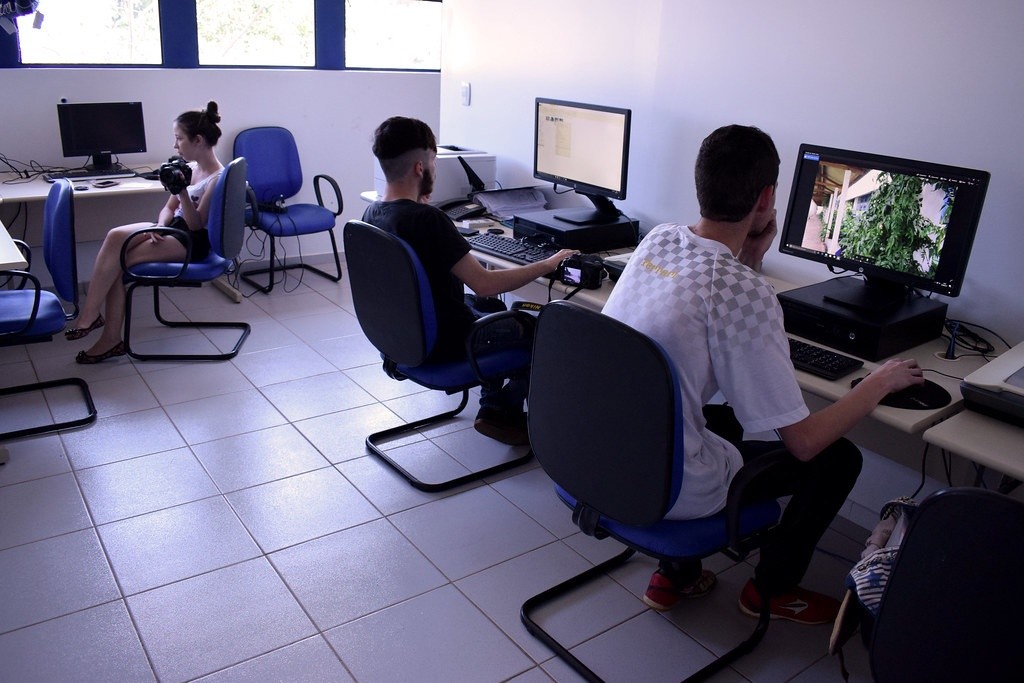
[232,127,343,293]
[519,299,781,683]
[0,183,97,441]
[829,487,1024,683]
[120,156,251,361]
[344,221,533,492]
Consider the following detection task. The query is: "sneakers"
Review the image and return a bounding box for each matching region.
[643,570,716,611]
[739,578,839,624]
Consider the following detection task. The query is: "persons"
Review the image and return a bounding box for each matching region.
[602,124,924,626]
[0,0,39,65]
[66,101,225,364]
[361,116,580,445]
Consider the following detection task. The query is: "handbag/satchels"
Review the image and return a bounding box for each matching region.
[844,495,923,614]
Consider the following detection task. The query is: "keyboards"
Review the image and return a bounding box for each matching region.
[42,169,135,183]
[467,233,557,265]
[788,337,864,381]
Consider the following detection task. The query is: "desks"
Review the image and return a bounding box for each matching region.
[922,404,1024,481]
[360,191,378,203]
[0,162,170,297]
[465,216,995,435]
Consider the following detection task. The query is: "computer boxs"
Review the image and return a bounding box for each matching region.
[775,275,948,363]
[513,206,640,254]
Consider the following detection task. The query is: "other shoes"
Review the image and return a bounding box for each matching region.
[66,314,106,340]
[75,341,128,364]
[474,411,529,446]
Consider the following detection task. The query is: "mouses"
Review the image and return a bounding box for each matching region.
[153,169,161,174]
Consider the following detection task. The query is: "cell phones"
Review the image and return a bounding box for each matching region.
[92,181,120,188]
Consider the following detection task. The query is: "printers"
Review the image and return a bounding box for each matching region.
[374,145,498,205]
[958,339,1024,430]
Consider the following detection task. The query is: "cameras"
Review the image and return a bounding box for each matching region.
[556,254,605,290]
[159,156,192,188]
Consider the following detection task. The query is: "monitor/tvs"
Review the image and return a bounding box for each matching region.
[57,102,147,172]
[532,98,631,225]
[779,144,991,317]
